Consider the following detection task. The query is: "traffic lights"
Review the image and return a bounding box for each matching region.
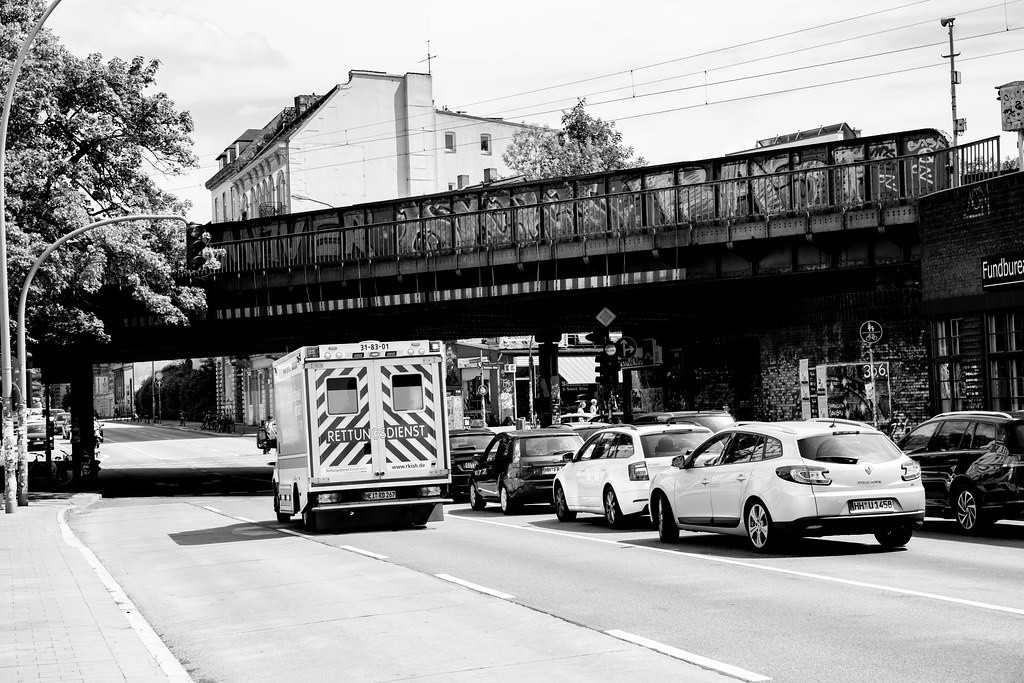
[595,355,608,386]
[185,222,206,271]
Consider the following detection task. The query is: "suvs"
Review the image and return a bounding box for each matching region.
[894,409,1024,536]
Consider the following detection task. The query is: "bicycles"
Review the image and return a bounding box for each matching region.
[178,413,187,427]
[198,414,235,434]
[28,448,74,486]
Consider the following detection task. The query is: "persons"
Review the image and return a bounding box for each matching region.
[259,416,277,454]
[578,399,598,422]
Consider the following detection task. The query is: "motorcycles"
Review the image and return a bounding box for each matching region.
[71,434,103,487]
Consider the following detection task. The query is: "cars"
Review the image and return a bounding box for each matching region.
[551,421,728,530]
[12,405,105,452]
[446,424,499,505]
[548,407,734,455]
[466,427,589,517]
[647,417,927,557]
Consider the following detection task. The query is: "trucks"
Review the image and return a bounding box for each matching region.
[257,337,454,532]
